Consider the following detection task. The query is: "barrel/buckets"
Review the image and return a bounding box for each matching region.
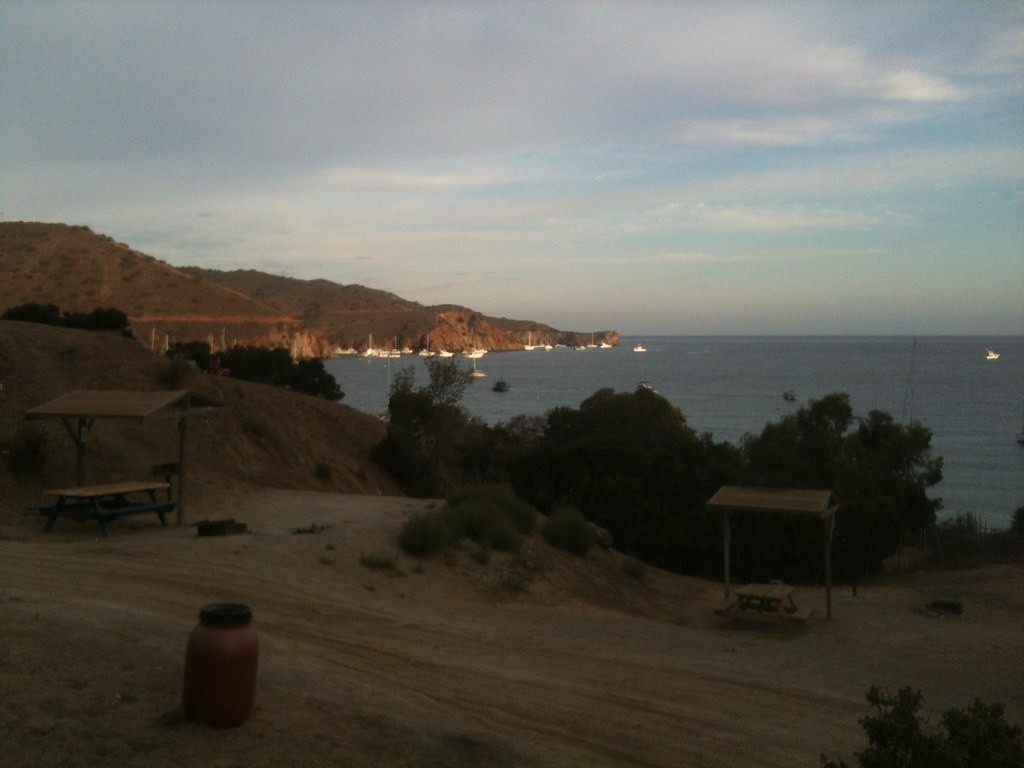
[183,600,257,726]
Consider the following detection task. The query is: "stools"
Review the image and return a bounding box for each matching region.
[734,583,799,615]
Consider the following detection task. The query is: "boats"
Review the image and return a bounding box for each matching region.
[337,329,647,379]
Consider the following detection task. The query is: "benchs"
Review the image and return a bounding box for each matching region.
[42,481,169,536]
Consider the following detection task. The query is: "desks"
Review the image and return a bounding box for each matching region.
[21,389,191,525]
